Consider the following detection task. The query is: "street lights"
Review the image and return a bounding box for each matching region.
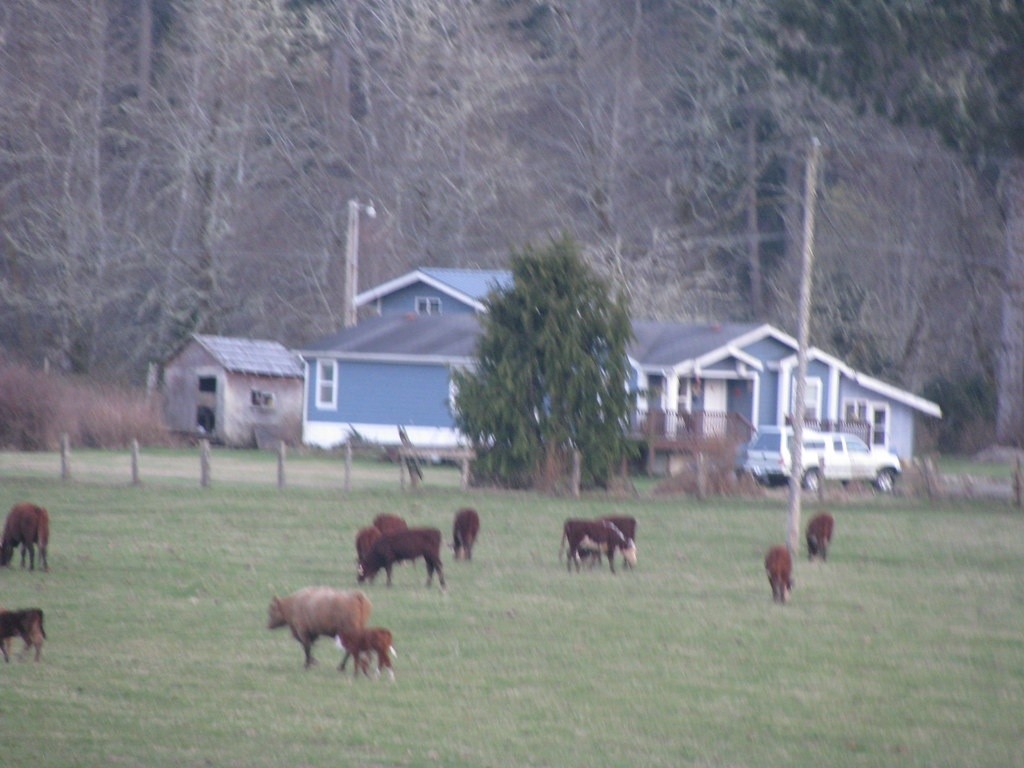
[342,200,377,334]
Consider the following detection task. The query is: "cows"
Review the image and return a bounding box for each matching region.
[0,501,51,571]
[329,625,397,682]
[452,508,479,561]
[764,542,795,600]
[0,607,47,664]
[268,586,371,672]
[356,513,447,588]
[806,511,835,560]
[559,516,638,575]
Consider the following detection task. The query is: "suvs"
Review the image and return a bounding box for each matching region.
[745,424,903,496]
